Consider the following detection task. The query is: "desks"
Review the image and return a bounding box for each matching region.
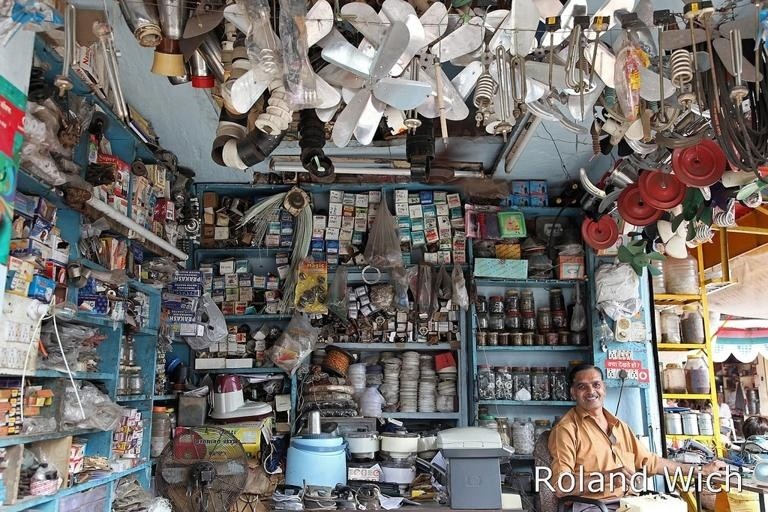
[692,469,768,512]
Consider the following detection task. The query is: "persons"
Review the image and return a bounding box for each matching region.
[545,361,728,512]
[741,414,768,440]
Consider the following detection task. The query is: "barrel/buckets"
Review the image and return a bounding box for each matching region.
[284,435,353,490]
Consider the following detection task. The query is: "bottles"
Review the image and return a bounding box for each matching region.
[478,416,550,455]
[152,405,178,457]
[307,410,320,434]
[119,0,230,90]
[651,250,716,394]
[614,44,648,121]
[477,358,584,401]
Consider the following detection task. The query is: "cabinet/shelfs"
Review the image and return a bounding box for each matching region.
[0,16,726,512]
